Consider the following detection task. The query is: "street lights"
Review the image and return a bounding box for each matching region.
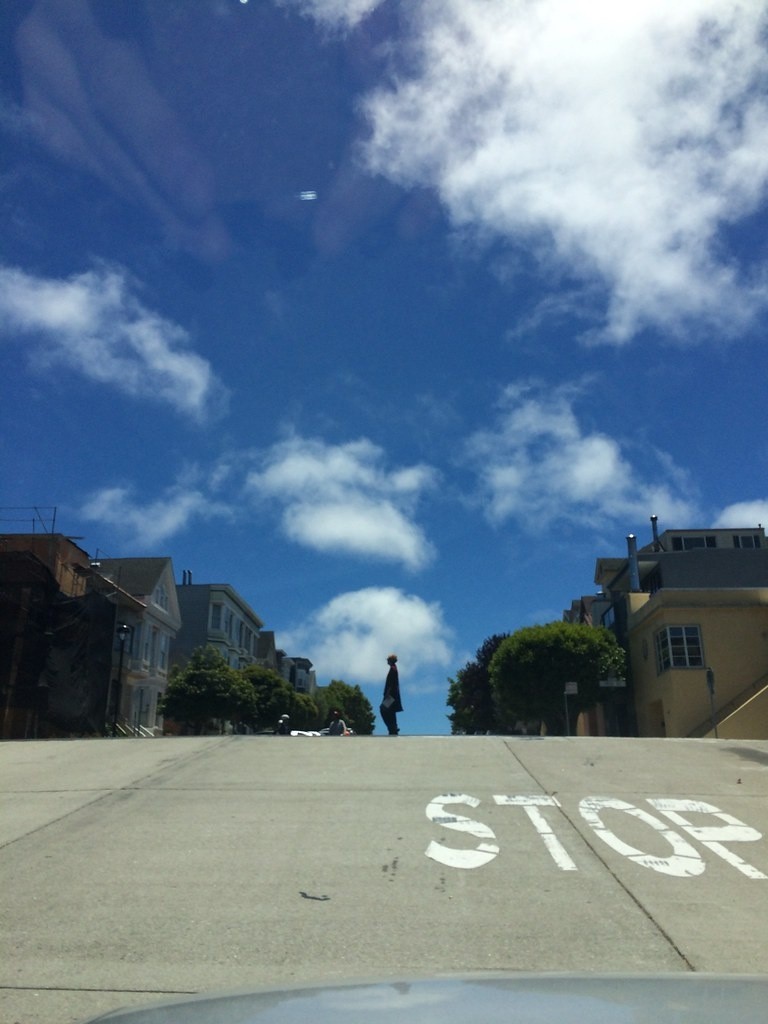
[113,623,132,737]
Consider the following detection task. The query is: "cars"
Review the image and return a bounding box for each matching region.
[256,726,358,737]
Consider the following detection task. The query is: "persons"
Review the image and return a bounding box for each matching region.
[380,657,404,736]
[329,711,346,736]
[275,714,291,735]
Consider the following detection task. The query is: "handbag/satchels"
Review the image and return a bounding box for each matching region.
[382,695,396,709]
